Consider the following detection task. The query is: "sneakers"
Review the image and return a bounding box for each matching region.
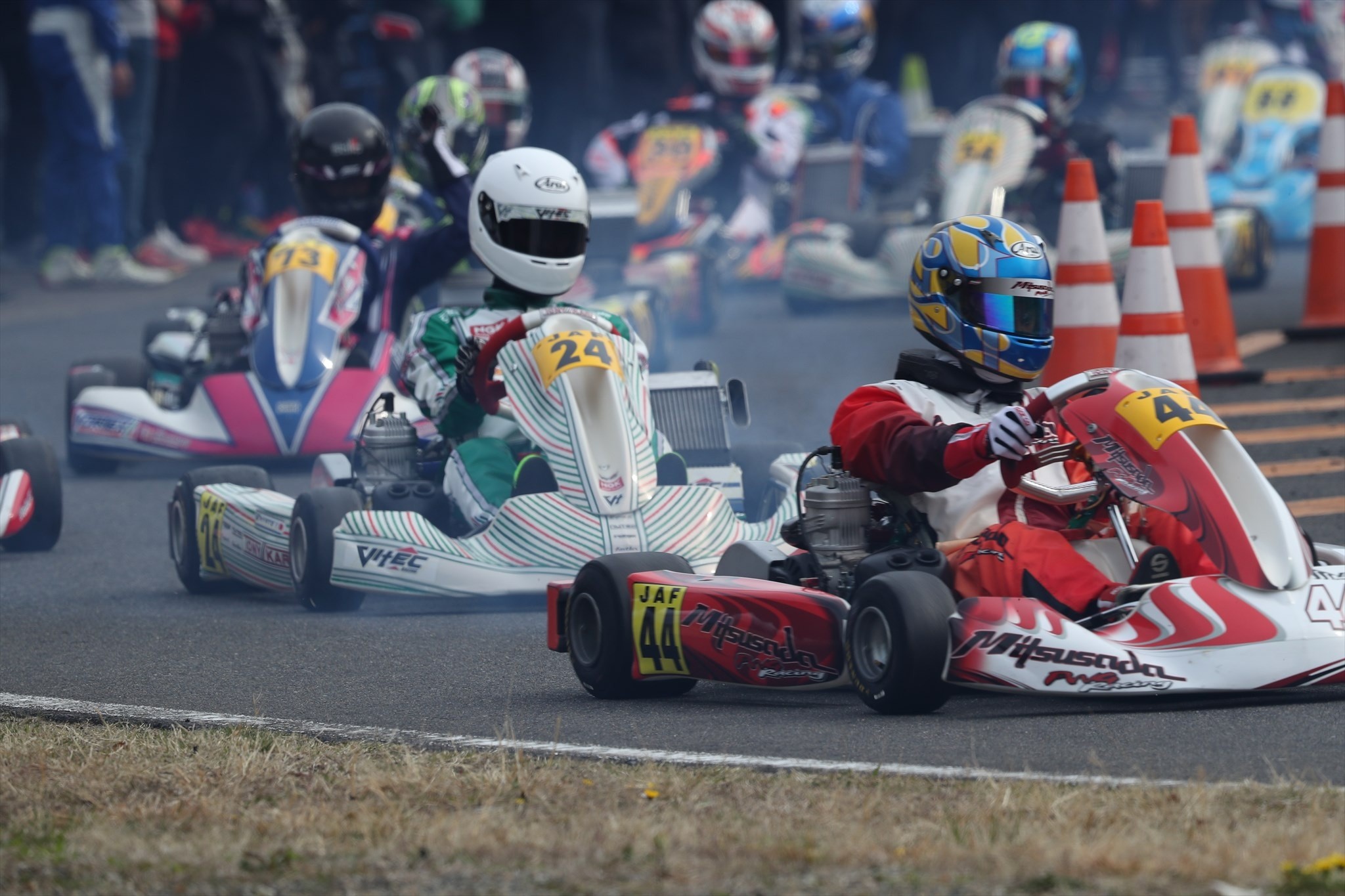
[42,238,173,285]
[1094,545,1181,624]
[134,228,210,275]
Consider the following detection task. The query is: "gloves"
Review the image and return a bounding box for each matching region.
[456,336,498,404]
[990,406,1046,461]
[418,102,469,181]
[725,119,759,160]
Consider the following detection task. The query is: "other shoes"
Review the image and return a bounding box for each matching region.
[512,454,559,497]
[181,217,259,261]
[241,210,298,241]
[657,451,688,485]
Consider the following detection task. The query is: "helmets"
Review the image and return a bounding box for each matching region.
[910,215,1054,383]
[295,104,393,231]
[691,0,778,98]
[787,0,877,87]
[450,48,533,148]
[397,73,488,183]
[467,147,589,296]
[995,20,1084,111]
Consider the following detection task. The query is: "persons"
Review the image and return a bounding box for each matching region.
[20,0,313,285]
[997,21,1120,248]
[144,104,474,402]
[387,146,689,532]
[832,214,1223,631]
[388,48,532,220]
[585,0,905,253]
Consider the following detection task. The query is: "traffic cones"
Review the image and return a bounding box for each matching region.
[1039,160,1120,388]
[1156,114,1265,387]
[1280,77,1345,340]
[1112,199,1200,399]
[898,53,947,138]
